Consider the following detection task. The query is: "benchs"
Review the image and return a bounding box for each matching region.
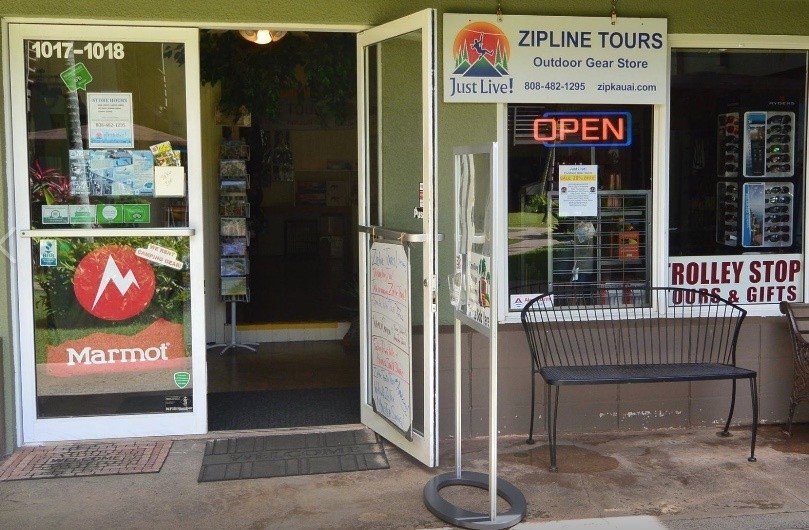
[777,299,809,438]
[520,286,762,472]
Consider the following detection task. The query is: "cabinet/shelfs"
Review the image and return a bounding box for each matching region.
[517,188,653,306]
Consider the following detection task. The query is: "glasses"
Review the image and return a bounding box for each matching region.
[766,164,790,172]
[719,164,738,175]
[716,115,739,124]
[718,182,738,193]
[767,115,791,124]
[720,153,738,163]
[722,126,739,135]
[766,196,790,204]
[767,144,789,153]
[719,195,738,203]
[767,124,791,133]
[720,144,739,152]
[764,225,789,232]
[718,224,738,232]
[720,204,738,212]
[719,214,738,221]
[722,135,739,144]
[765,214,789,223]
[766,153,791,162]
[765,234,789,242]
[766,206,788,213]
[765,186,789,194]
[767,135,791,143]
[718,234,737,241]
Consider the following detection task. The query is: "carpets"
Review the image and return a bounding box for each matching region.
[0,441,174,481]
[197,427,391,483]
[117,386,360,433]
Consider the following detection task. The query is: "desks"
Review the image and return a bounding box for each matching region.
[258,204,354,273]
